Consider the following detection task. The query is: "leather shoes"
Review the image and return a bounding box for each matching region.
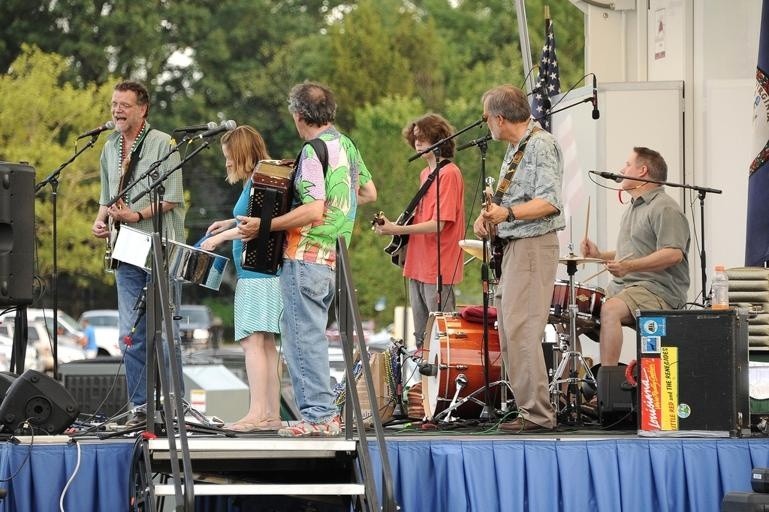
[497,417,551,433]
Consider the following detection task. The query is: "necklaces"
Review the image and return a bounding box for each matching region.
[117,118,148,226]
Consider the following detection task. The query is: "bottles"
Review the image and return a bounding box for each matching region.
[711,266,730,310]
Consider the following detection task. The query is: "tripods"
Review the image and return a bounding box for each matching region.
[549,274,598,422]
[434,361,514,424]
[101,187,236,439]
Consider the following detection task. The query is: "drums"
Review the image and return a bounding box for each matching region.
[552,281,606,328]
[112,224,230,290]
[422,312,500,420]
[501,343,559,421]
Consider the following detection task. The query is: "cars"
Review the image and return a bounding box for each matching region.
[322,323,370,347]
[171,304,224,351]
[368,325,394,352]
[0,307,121,372]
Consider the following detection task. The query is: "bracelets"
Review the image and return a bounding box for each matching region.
[136,212,145,224]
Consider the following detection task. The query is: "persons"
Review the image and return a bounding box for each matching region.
[91,80,187,438]
[200,124,285,434]
[70,319,98,362]
[370,112,466,431]
[236,82,379,438]
[473,83,565,433]
[52,324,74,345]
[553,147,692,419]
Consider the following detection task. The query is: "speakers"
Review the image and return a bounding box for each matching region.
[0,160,36,307]
[636,310,750,438]
[0,370,18,405]
[1,369,80,436]
[596,366,633,430]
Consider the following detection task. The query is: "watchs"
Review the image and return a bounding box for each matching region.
[506,206,515,223]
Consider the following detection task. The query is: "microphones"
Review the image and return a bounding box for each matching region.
[592,170,624,183]
[539,68,552,110]
[77,121,115,139]
[199,119,238,137]
[173,121,218,132]
[411,358,436,376]
[592,76,599,121]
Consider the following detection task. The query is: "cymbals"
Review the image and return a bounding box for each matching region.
[559,256,605,262]
[459,239,493,260]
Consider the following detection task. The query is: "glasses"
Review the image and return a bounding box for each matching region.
[111,102,144,109]
[413,135,428,143]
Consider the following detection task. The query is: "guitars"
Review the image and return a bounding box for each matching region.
[372,210,417,267]
[482,179,504,282]
[105,156,132,269]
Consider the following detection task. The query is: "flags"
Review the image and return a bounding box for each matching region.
[530,18,562,133]
[744,1,769,268]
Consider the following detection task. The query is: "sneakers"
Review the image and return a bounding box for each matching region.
[125,412,147,425]
[221,418,281,434]
[277,418,340,437]
